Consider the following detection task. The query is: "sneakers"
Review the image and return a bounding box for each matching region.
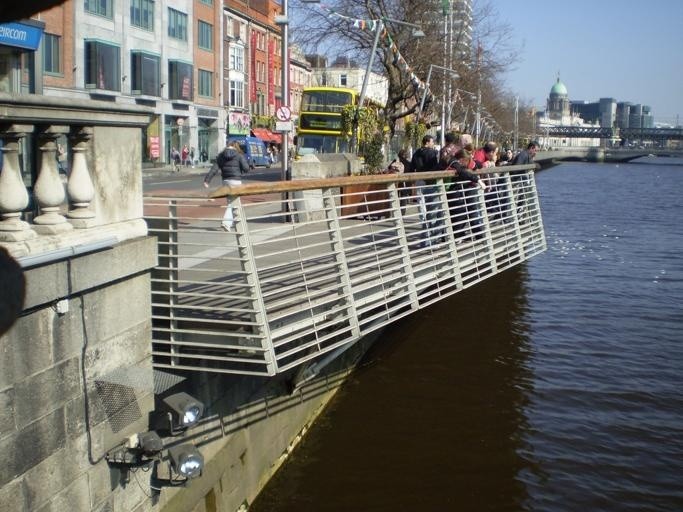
[220,224,232,233]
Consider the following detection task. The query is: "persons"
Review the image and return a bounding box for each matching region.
[203,141,249,232]
[388,133,540,255]
[170,145,196,171]
[267,146,295,164]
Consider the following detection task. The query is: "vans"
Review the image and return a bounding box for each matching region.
[227,136,271,168]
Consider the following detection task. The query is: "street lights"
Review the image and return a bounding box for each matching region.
[415,63,462,127]
[447,88,514,142]
[353,16,427,126]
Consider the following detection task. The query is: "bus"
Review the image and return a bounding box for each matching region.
[294,87,390,161]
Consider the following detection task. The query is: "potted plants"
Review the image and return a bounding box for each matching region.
[340,127,416,221]
